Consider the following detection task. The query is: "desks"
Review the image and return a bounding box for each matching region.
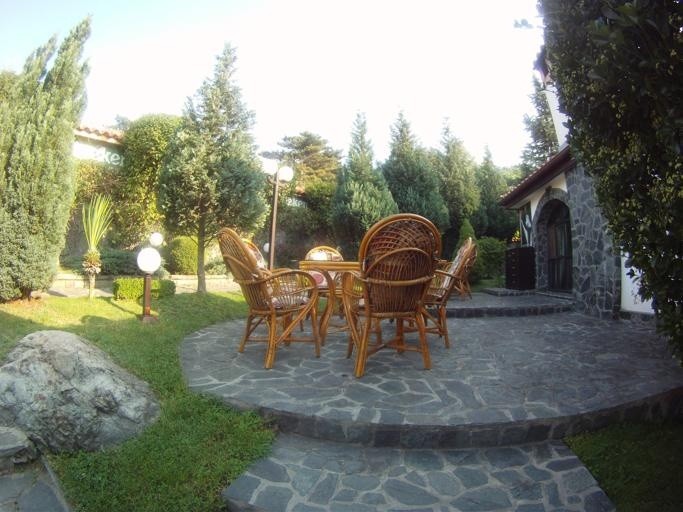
[299,261,448,357]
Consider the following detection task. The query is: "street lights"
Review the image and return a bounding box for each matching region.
[266,165,296,273]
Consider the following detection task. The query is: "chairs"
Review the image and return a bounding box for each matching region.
[304,244,350,346]
[454,246,478,302]
[396,235,472,355]
[342,214,442,377]
[217,226,322,369]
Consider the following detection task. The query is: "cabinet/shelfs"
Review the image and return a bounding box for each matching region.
[505,246,535,289]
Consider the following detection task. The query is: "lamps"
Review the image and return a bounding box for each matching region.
[136,248,162,322]
